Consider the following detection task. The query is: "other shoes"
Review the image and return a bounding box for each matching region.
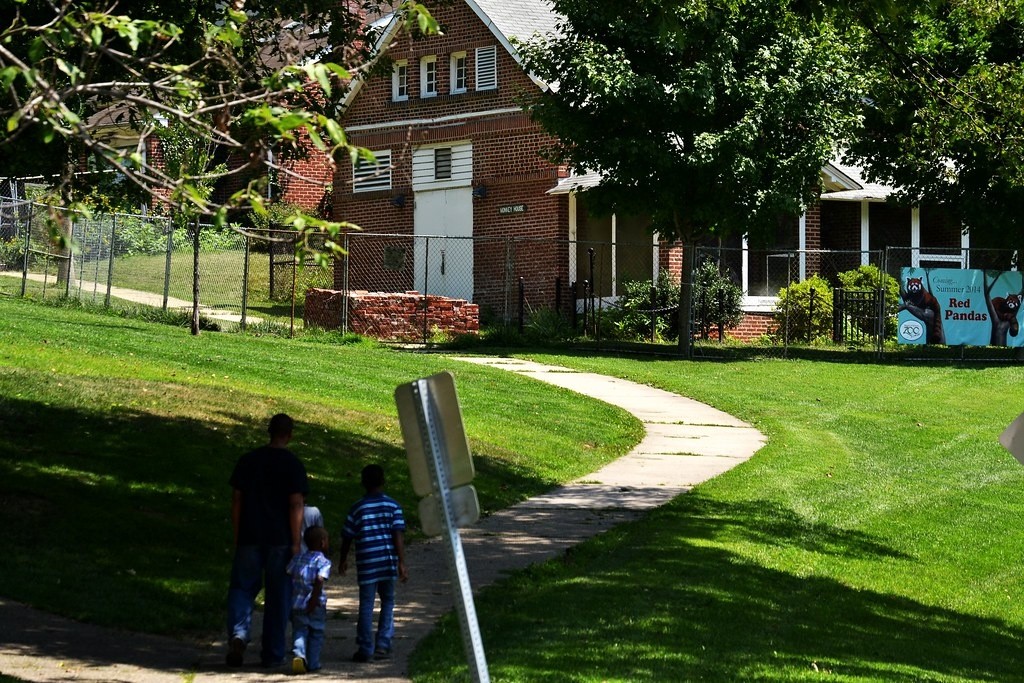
[373,650,396,659]
[225,635,246,667]
[350,651,375,663]
[260,656,288,667]
[290,656,308,674]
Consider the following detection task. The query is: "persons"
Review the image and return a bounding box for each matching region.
[339,466,411,662]
[225,415,331,672]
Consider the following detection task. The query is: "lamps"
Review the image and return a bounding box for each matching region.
[390,193,406,207]
[472,182,486,199]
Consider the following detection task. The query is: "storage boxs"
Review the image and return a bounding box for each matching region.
[302,286,479,339]
